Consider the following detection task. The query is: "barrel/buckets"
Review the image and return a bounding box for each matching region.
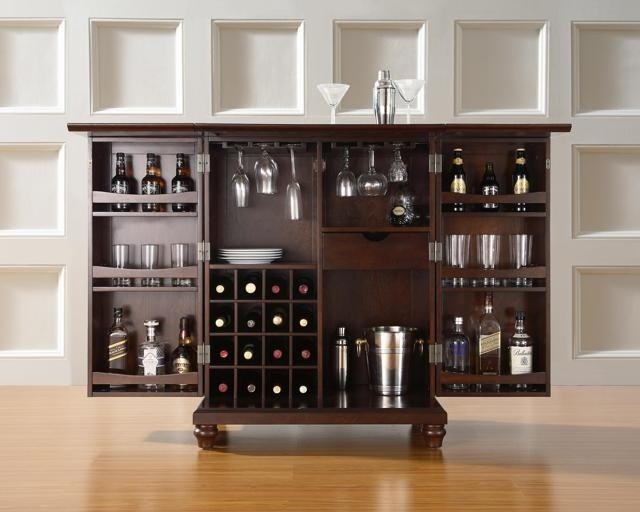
[353,324,426,398]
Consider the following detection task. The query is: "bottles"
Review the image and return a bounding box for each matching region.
[105,307,130,387]
[475,291,502,392]
[166,317,198,391]
[328,325,356,390]
[508,312,534,391]
[385,183,421,226]
[479,161,501,212]
[110,153,131,212]
[171,153,194,211]
[141,153,162,211]
[442,316,472,390]
[446,148,468,212]
[136,319,166,389]
[209,272,317,410]
[510,148,532,212]
[372,70,396,125]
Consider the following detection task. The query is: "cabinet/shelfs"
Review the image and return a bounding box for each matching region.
[435,123,571,398]
[66,124,204,398]
[193,124,447,451]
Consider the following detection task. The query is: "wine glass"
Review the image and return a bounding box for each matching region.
[335,144,357,198]
[387,143,409,183]
[231,147,250,208]
[253,143,279,195]
[316,83,351,124]
[283,144,305,220]
[357,143,389,197]
[391,78,425,124]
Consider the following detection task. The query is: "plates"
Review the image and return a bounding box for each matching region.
[215,247,283,265]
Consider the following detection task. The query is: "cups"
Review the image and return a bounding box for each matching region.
[475,233,502,287]
[445,234,471,286]
[169,243,189,286]
[140,244,159,286]
[508,233,535,287]
[111,244,129,286]
